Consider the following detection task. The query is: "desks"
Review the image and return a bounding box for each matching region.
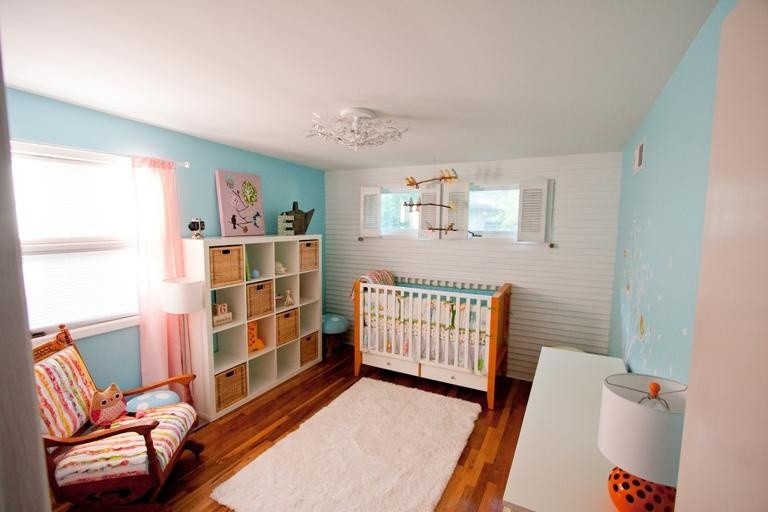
[501,344,629,512]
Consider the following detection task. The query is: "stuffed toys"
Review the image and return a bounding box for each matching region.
[88,382,146,430]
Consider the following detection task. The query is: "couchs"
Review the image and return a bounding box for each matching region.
[30,323,205,512]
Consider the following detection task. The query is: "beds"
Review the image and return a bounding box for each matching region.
[354,275,512,410]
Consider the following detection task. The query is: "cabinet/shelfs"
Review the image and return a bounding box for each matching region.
[180,234,323,423]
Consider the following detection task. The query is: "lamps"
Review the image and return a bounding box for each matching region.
[596,372,688,512]
[306,107,410,152]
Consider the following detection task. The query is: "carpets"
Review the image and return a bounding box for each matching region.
[207,375,483,512]
